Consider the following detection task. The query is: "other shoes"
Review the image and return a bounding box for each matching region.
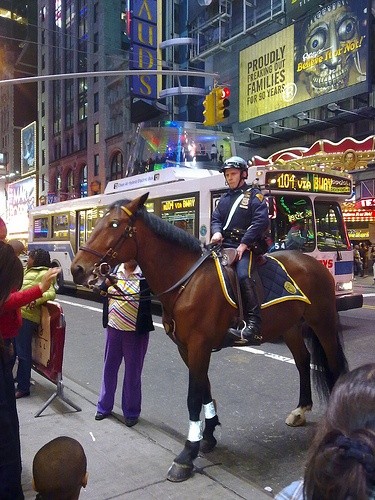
[14,390,30,398]
[95,410,111,419]
[124,415,138,427]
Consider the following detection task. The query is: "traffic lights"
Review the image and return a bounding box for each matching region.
[215,87,230,121]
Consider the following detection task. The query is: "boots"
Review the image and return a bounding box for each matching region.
[227,305,261,342]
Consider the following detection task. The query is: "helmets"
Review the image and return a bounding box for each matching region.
[219,156,248,173]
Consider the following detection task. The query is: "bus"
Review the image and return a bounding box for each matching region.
[25,165,364,318]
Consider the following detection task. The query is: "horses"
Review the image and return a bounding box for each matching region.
[71,191,351,484]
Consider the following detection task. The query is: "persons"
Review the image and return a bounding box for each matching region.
[352,241,375,277]
[211,157,270,346]
[15,248,56,399]
[29,436,88,500]
[131,144,224,175]
[284,219,311,251]
[272,362,375,500]
[0,240,25,500]
[8,238,29,274]
[0,268,61,372]
[96,261,155,428]
[303,428,375,500]
